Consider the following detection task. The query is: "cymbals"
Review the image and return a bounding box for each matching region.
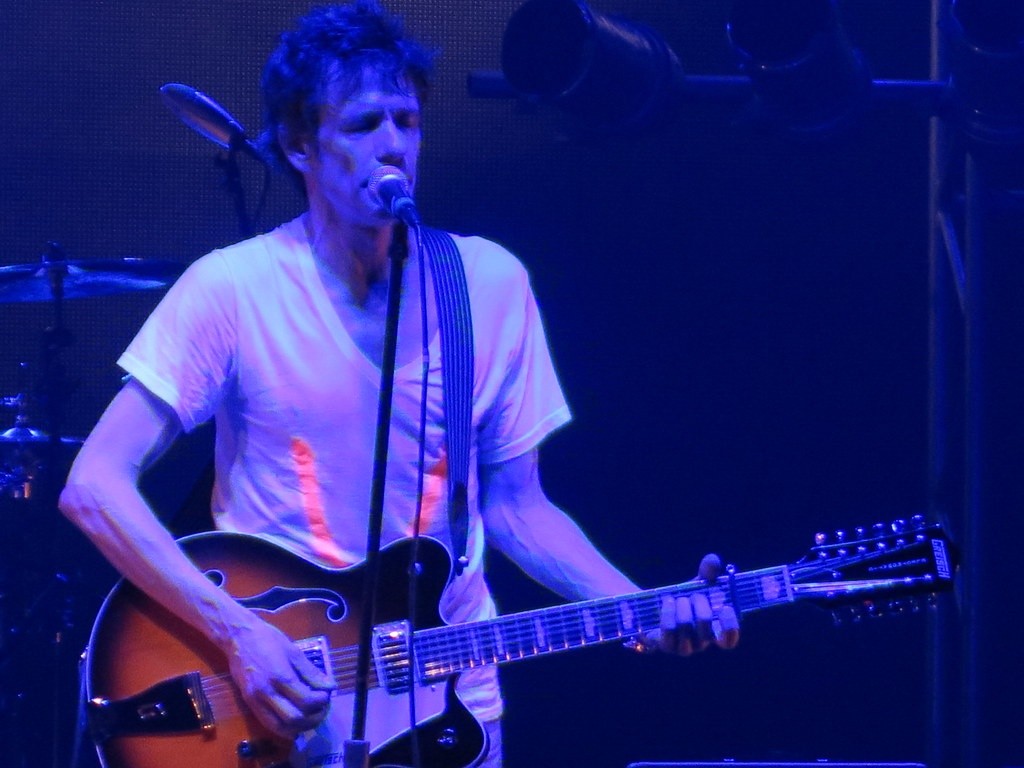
[1,424,88,447]
[0,259,166,309]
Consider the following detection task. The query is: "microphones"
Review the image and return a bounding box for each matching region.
[369,165,421,229]
[162,84,258,160]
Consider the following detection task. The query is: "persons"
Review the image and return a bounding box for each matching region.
[58,4,739,768]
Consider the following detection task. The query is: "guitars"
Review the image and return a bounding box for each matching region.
[73,512,965,768]
